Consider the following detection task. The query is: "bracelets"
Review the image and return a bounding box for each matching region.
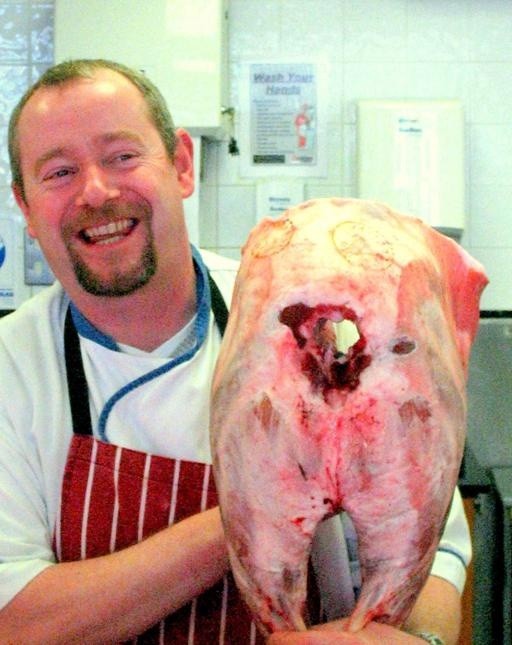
[410,629,444,644]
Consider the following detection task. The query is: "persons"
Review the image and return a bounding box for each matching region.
[0,57,473,645]
